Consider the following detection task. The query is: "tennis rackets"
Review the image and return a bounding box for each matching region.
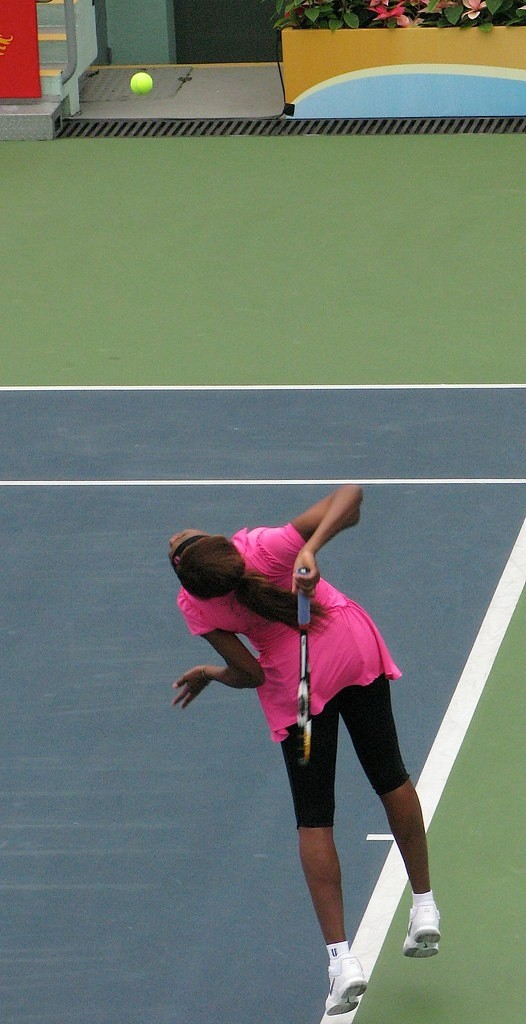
[296,566,312,767]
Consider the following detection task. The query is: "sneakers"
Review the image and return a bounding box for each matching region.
[402,905,441,959]
[325,954,368,1016]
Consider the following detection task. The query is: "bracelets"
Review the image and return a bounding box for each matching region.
[200,664,208,685]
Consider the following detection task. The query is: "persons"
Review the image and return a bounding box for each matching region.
[167,484,441,1016]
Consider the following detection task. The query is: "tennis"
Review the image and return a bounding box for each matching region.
[129,71,154,96]
